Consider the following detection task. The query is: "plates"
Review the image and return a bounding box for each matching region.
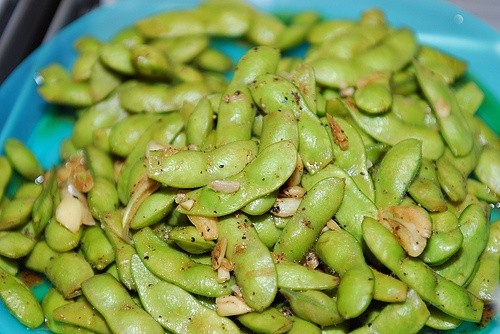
[0,0,500,334]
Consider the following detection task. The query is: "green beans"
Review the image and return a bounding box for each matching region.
[0,0,500,334]
